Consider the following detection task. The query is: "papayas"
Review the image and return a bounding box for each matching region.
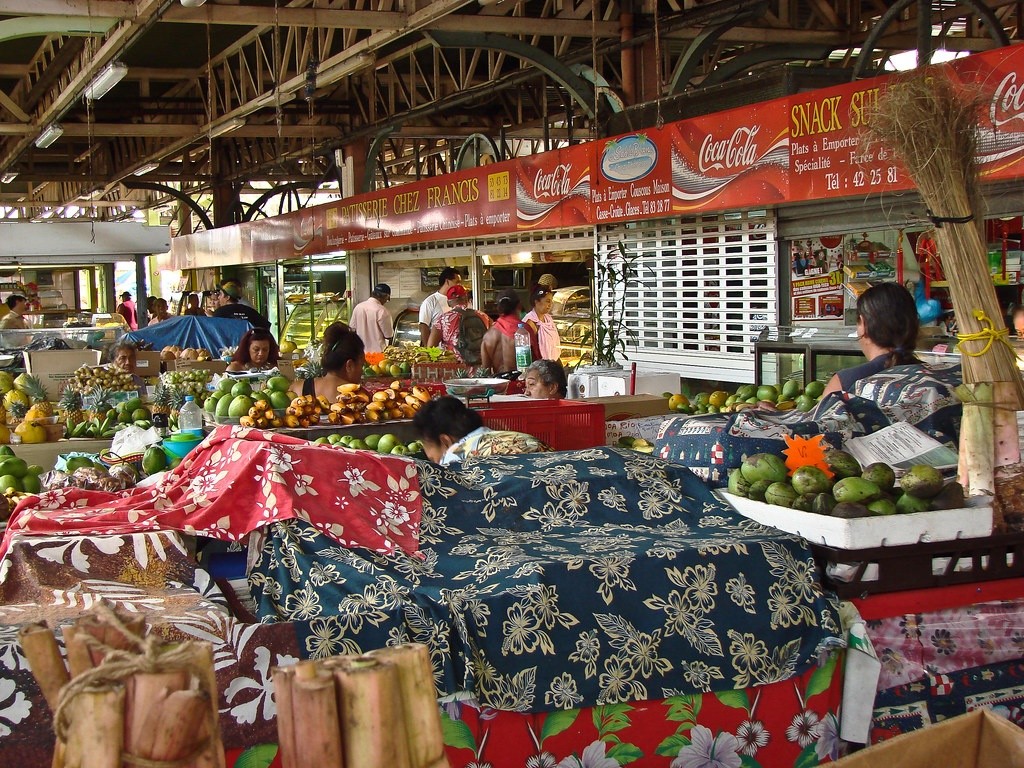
[106,398,151,422]
[0,444,167,495]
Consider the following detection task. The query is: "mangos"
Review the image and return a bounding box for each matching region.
[668,379,826,412]
[203,376,299,418]
[362,359,411,378]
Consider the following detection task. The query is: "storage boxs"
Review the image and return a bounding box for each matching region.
[1,349,1023,767]
[844,246,893,295]
[1000,248,1024,284]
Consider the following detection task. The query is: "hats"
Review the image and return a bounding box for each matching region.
[495,288,518,304]
[445,284,466,300]
[371,283,392,301]
[119,291,132,297]
[215,280,242,302]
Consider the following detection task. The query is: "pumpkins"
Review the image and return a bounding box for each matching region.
[159,344,210,360]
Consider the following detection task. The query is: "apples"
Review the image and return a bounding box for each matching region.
[313,433,424,454]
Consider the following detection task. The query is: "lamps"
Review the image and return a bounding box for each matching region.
[308,52,376,89]
[209,119,247,139]
[134,160,160,178]
[78,188,105,201]
[87,62,128,102]
[34,124,62,150]
[1,169,18,184]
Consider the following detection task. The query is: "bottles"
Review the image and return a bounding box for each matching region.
[153,412,168,438]
[226,356,231,362]
[514,324,531,371]
[179,396,203,436]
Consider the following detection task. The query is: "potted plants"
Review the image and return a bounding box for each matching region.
[564,244,646,369]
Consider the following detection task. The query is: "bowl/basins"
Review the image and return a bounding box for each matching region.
[0,355,15,367]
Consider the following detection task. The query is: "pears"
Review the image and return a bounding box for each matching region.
[167,368,213,401]
[67,366,142,393]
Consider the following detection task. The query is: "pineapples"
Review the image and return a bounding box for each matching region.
[151,385,187,419]
[17,374,113,423]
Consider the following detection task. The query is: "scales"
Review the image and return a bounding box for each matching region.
[442,378,509,409]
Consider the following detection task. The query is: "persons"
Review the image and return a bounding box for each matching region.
[147,281,272,328]
[411,395,556,466]
[419,267,561,374]
[349,283,394,354]
[101,341,148,398]
[225,327,279,373]
[0,294,30,329]
[288,321,366,403]
[117,291,138,331]
[820,282,928,403]
[524,359,568,399]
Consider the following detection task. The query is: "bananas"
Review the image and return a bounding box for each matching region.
[240,383,431,429]
[385,346,459,365]
[3,487,26,518]
[66,416,151,438]
[616,436,654,451]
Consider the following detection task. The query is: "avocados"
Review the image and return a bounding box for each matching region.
[728,450,963,518]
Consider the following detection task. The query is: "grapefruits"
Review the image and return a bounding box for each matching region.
[0,370,48,443]
[278,341,304,359]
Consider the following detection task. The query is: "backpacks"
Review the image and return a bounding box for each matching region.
[453,307,488,365]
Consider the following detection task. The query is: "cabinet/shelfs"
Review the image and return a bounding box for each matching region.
[838,241,900,300]
[275,301,347,350]
